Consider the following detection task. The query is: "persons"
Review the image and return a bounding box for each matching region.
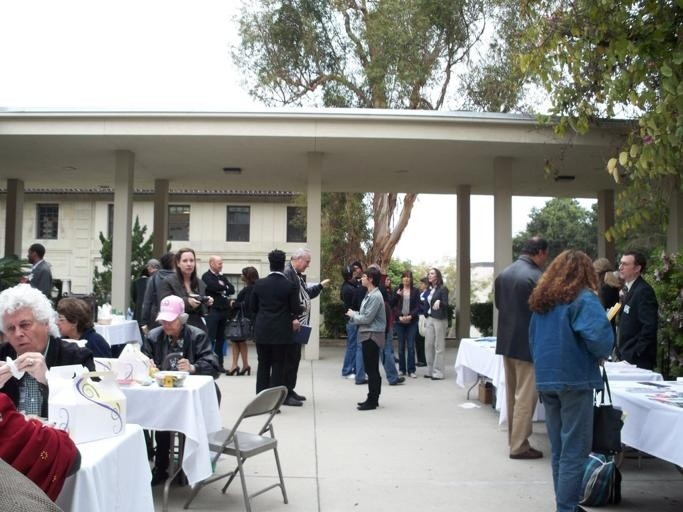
[593,257,621,362]
[340,259,449,410]
[202,255,235,373]
[285,247,331,406]
[250,248,300,414]
[614,251,658,370]
[141,294,222,486]
[132,253,175,335]
[493,236,550,459]
[525,250,616,512]
[225,265,260,376]
[152,246,215,334]
[0,243,114,511]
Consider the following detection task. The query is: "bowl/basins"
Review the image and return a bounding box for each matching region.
[157,370,191,389]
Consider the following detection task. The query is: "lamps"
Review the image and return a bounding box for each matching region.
[553,173,576,183]
[222,166,242,175]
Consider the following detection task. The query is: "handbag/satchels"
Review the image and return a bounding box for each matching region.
[418,314,429,338]
[223,301,255,342]
[591,361,625,460]
[49,364,128,444]
[578,450,624,508]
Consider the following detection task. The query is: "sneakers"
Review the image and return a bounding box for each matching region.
[149,468,188,488]
[392,377,406,386]
[398,370,443,380]
[355,378,369,385]
[343,372,356,379]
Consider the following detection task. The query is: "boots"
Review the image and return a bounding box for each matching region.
[356,393,379,411]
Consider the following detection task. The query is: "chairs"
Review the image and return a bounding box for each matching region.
[183,384,295,512]
[160,381,220,487]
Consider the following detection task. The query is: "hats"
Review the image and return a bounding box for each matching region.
[154,295,186,323]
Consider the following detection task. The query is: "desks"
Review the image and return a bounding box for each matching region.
[103,363,221,510]
[602,377,681,478]
[92,317,143,351]
[26,413,158,511]
[492,350,665,468]
[450,338,503,408]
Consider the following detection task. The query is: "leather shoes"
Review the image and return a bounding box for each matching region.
[282,395,303,406]
[289,390,307,401]
[510,445,544,459]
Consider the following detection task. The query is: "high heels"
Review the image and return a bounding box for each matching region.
[237,366,251,377]
[225,367,240,377]
